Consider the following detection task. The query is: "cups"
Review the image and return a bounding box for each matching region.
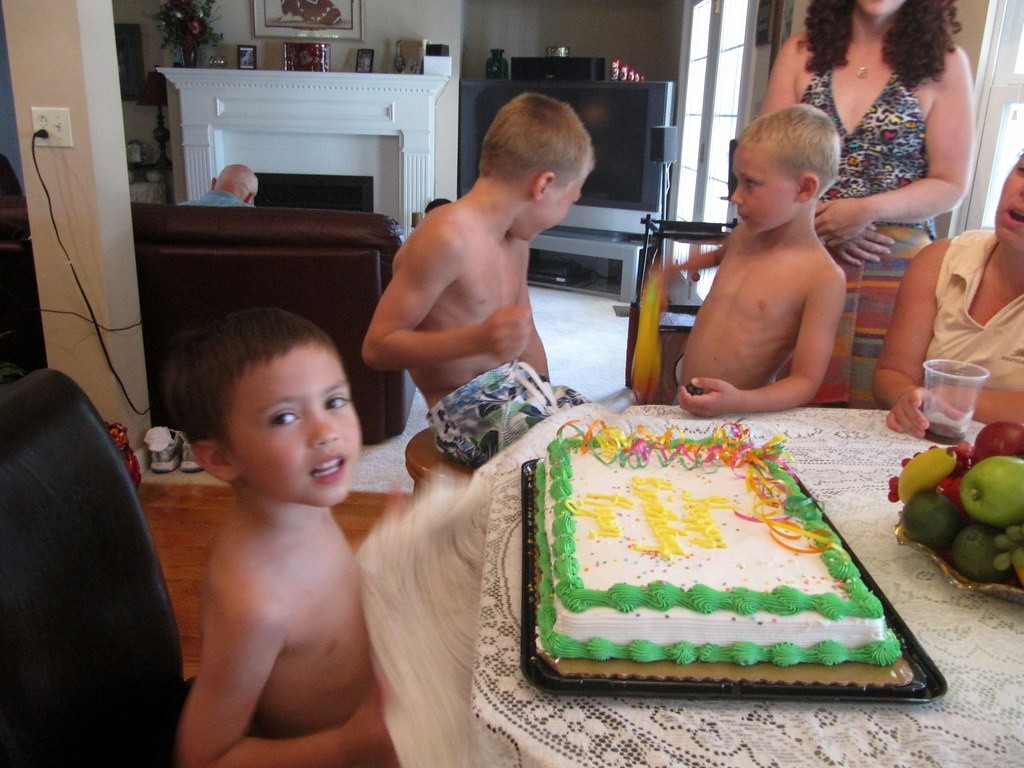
[922,360,990,445]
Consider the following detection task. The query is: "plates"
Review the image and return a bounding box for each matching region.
[894,513,1024,605]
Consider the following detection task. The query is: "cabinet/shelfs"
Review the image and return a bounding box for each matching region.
[527,228,658,303]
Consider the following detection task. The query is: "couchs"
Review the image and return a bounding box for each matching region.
[0,196,417,441]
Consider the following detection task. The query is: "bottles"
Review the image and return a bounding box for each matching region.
[485,48,508,82]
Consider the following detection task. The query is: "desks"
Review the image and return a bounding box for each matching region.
[354,407,1024,767]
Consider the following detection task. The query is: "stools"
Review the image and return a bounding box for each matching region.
[405,424,472,501]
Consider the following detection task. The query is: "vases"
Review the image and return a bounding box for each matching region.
[182,40,197,68]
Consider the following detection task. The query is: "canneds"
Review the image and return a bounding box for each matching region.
[609,58,640,82]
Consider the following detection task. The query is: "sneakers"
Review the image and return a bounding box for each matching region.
[181,442,203,470]
[150,429,180,471]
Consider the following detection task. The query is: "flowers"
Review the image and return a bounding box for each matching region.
[152,0,224,46]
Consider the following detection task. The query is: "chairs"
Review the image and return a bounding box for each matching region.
[627,306,694,407]
[0,368,193,768]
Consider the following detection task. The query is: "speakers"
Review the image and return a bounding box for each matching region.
[510,56,606,81]
[649,127,679,164]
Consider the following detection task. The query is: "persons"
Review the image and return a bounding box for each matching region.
[179,164,258,207]
[362,92,594,413]
[871,151,1024,439]
[662,103,848,417]
[166,308,399,768]
[759,0,975,410]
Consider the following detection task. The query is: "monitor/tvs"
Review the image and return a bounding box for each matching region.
[459,78,674,242]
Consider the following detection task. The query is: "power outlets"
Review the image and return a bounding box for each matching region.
[32,106,71,146]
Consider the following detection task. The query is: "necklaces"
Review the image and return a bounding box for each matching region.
[849,54,868,78]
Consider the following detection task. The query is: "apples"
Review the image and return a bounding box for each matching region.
[942,420,1024,528]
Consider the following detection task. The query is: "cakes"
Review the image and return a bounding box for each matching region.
[531,419,904,666]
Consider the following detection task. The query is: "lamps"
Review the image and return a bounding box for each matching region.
[134,69,172,170]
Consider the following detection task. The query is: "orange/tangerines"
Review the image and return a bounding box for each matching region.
[902,492,1014,587]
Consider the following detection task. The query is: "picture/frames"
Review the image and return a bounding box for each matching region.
[114,23,146,99]
[355,48,375,72]
[236,44,257,70]
[248,0,364,41]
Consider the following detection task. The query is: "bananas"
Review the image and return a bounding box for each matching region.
[898,447,956,503]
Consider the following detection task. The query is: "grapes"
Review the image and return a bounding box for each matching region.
[993,524,1024,572]
[887,441,974,503]
